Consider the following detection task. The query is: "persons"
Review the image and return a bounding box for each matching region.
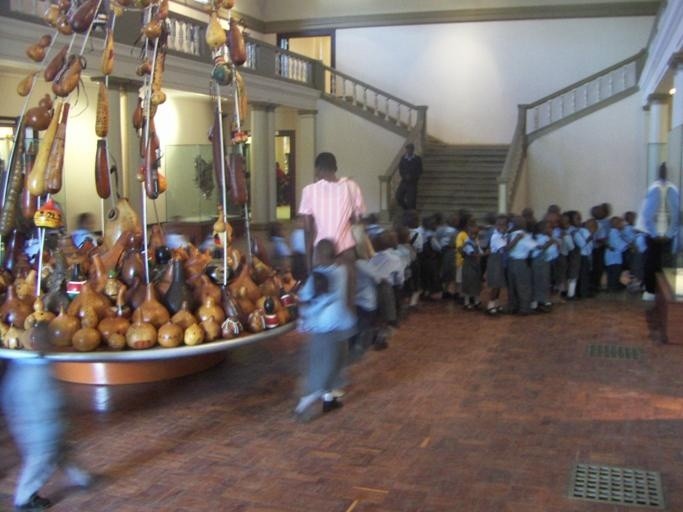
[0,320,96,512]
[359,202,647,351]
[290,238,357,424]
[296,150,366,276]
[633,163,668,302]
[395,141,424,211]
[664,181,682,258]
[13,192,292,279]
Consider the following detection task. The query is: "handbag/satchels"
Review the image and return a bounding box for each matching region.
[352,223,377,259]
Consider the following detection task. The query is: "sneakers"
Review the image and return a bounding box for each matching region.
[323,397,342,412]
[509,301,552,315]
[442,292,503,314]
